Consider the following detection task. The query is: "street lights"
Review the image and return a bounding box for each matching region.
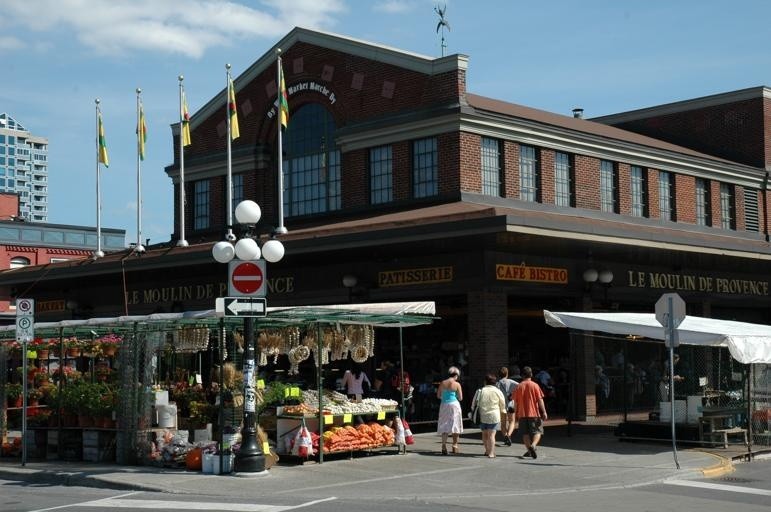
[212,200,286,472]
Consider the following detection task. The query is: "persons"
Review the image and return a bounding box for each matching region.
[341,361,372,400]
[436,367,463,454]
[626,363,640,408]
[471,374,506,458]
[494,366,519,446]
[512,366,548,459]
[658,354,686,400]
[371,358,395,402]
[535,368,556,393]
[597,364,611,400]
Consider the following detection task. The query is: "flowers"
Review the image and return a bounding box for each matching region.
[0,336,221,462]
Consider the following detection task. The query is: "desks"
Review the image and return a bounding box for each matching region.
[698,406,749,448]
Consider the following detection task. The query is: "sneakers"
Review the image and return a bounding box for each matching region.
[522,444,539,461]
[504,434,513,446]
[442,446,458,455]
[483,450,496,458]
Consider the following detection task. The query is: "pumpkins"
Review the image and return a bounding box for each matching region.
[187,447,202,469]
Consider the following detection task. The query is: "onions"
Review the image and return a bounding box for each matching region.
[322,426,360,452]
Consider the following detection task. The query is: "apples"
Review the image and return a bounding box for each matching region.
[285,403,319,414]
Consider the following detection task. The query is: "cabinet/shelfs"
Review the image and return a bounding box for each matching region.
[277,403,405,463]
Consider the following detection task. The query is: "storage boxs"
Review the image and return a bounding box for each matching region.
[258,414,277,429]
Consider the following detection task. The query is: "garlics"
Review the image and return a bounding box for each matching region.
[302,389,398,415]
[172,325,374,375]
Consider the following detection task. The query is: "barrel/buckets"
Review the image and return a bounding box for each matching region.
[158,404,176,428]
[213,455,228,475]
[201,453,213,473]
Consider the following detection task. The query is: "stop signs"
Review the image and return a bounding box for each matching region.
[227,260,267,299]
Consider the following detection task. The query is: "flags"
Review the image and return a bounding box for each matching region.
[181,90,192,147]
[230,79,240,142]
[279,60,289,134]
[99,112,110,168]
[139,99,148,161]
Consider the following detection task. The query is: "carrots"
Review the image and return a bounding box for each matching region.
[357,423,395,449]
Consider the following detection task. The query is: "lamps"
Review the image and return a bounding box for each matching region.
[582,269,614,310]
[343,275,371,304]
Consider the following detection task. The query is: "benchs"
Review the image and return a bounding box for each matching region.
[715,427,750,448]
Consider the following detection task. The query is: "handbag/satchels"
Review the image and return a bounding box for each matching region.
[506,398,516,413]
[468,405,479,426]
